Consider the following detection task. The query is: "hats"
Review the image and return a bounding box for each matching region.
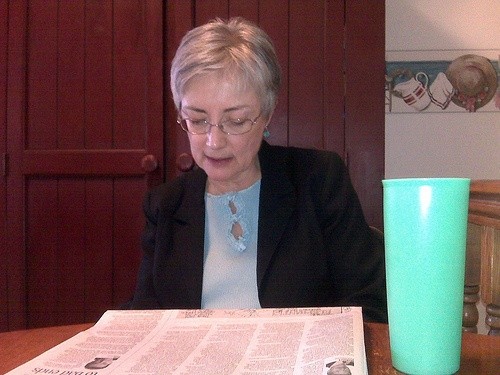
[446,53,496,112]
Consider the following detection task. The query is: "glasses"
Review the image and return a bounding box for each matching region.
[177,108,265,135]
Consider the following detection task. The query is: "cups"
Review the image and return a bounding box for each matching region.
[381,177,474,375]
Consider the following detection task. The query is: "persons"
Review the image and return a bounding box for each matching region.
[131,17,388,323]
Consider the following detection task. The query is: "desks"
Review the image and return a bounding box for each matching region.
[0,319,500,375]
